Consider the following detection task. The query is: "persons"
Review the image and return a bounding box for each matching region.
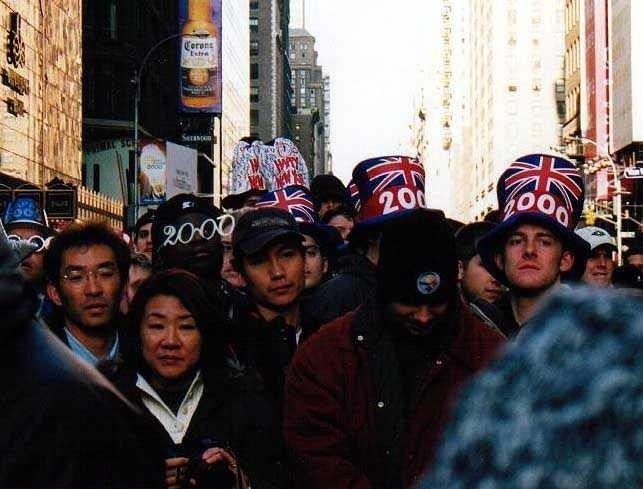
[1,171,643,489]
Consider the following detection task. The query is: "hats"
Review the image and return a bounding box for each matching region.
[223,137,456,302]
[477,154,590,284]
[151,192,222,235]
[0,198,58,268]
[574,226,617,255]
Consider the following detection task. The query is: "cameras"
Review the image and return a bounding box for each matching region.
[166,438,237,489]
[611,262,642,288]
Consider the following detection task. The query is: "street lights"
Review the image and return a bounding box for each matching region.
[580,138,623,268]
[133,27,210,235]
